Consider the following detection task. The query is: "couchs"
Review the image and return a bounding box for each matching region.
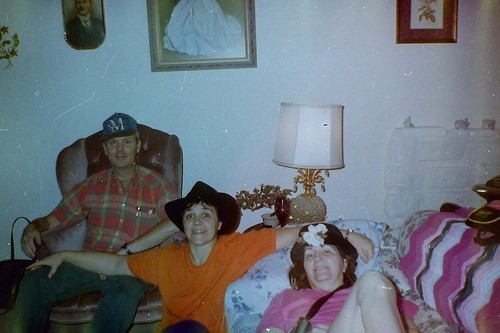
[244,210,500,333]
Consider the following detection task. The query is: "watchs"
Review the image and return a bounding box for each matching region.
[345,228,354,240]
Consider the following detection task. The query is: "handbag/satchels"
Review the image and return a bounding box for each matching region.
[0,216,33,311]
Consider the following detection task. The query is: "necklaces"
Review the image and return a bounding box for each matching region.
[189,244,213,265]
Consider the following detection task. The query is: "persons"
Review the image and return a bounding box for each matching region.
[256,223,423,333]
[26,181,375,333]
[10,113,179,333]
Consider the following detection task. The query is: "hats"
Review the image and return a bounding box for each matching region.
[100,112,138,141]
[290,222,358,267]
[164,181,241,234]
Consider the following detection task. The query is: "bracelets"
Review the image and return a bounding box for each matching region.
[29,222,40,229]
[121,242,132,255]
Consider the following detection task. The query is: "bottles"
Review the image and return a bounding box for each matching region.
[292,318,310,333]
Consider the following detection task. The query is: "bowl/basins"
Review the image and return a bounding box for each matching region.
[261,213,279,227]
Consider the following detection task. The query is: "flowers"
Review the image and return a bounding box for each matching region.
[297,224,328,247]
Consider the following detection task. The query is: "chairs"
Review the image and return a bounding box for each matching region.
[36,124,184,327]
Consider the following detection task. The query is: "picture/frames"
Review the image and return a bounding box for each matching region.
[396,0,459,44]
[146,0,257,72]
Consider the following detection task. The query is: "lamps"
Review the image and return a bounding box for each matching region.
[272,102,344,224]
